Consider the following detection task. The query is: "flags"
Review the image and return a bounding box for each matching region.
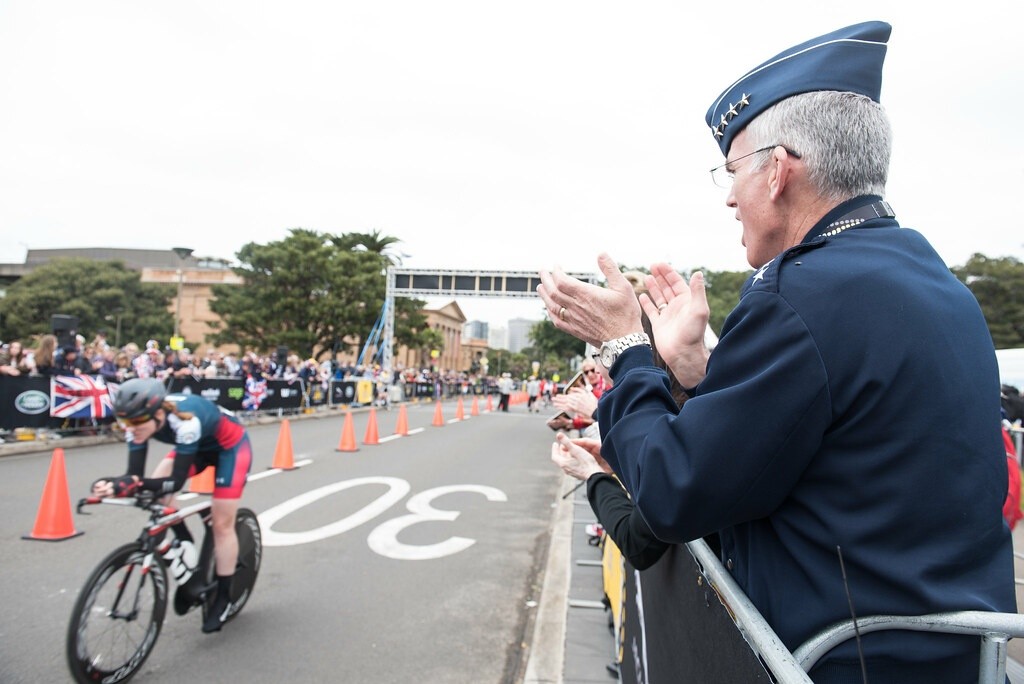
[50,374,112,418]
[242,374,268,410]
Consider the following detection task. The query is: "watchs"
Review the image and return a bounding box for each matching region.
[600,332,651,370]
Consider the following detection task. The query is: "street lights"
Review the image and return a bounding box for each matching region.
[170,248,193,336]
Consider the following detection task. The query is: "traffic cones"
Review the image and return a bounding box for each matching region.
[181,467,215,495]
[485,394,494,411]
[470,396,481,417]
[433,400,445,427]
[361,409,382,446]
[333,412,360,453]
[391,404,410,437]
[267,420,300,471]
[22,446,84,542]
[455,397,466,421]
[508,391,529,404]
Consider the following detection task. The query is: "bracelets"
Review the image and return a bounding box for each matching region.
[569,419,572,424]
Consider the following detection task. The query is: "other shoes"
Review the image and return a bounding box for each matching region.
[160,535,193,569]
[202,592,234,632]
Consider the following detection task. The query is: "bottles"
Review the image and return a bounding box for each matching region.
[173,540,199,570]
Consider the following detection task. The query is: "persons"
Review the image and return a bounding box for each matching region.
[91,378,253,634]
[547,270,690,575]
[394,363,496,386]
[527,374,557,412]
[0,334,381,381]
[498,371,516,411]
[536,20,1017,684]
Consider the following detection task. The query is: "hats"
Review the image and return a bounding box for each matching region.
[705,21,892,159]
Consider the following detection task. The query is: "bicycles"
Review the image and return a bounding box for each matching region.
[65,478,262,684]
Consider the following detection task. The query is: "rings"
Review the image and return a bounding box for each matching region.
[560,308,566,321]
[657,303,667,314]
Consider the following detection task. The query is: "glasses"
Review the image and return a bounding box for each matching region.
[115,406,161,427]
[584,367,595,375]
[710,144,801,190]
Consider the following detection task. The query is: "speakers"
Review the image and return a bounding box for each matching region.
[50,314,78,351]
[277,346,288,367]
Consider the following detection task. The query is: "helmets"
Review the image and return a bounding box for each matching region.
[109,378,162,419]
